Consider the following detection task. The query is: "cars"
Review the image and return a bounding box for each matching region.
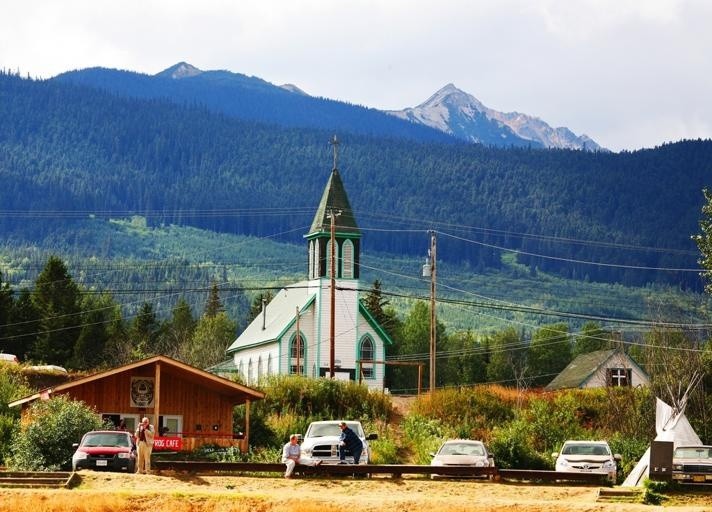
[551,438,622,485]
[672,444,712,490]
[69,430,136,474]
[429,439,497,481]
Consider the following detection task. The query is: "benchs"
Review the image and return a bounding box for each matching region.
[154,461,609,486]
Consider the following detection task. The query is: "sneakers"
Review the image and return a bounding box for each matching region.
[284,476,293,479]
[337,461,348,464]
[136,470,151,474]
[316,459,324,467]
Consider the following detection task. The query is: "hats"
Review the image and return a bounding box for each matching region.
[338,421,346,427]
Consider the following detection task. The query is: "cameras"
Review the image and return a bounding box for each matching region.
[140,423,144,426]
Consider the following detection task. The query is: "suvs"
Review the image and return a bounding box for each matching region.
[293,418,379,479]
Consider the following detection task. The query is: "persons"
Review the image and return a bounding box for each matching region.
[337,421,363,477]
[282,434,324,478]
[116,421,129,433]
[134,416,155,474]
